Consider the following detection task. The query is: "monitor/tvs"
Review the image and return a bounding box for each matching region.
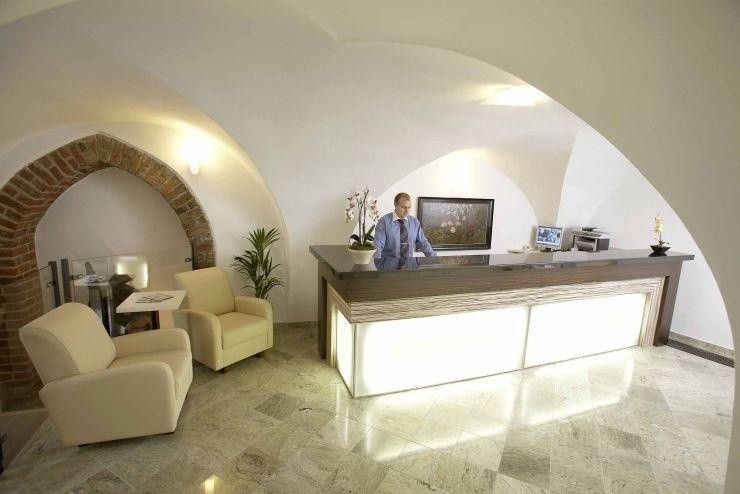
[534,225,563,251]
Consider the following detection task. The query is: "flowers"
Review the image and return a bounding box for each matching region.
[344,186,381,249]
[654,215,668,245]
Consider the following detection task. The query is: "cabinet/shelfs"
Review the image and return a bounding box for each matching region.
[573,231,610,252]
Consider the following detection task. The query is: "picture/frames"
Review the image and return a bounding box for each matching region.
[416,196,495,249]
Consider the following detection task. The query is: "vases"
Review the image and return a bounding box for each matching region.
[651,244,671,253]
[347,246,376,265]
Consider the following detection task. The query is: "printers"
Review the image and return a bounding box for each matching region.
[569,229,615,251]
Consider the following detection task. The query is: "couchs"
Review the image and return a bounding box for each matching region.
[19,300,195,448]
[173,266,273,372]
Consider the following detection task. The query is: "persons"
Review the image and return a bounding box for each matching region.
[374,256,426,270]
[370,193,438,256]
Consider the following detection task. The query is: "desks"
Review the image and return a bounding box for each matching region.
[73,272,132,338]
[114,289,187,329]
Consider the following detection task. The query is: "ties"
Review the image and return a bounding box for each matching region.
[398,219,409,257]
[397,258,406,270]
